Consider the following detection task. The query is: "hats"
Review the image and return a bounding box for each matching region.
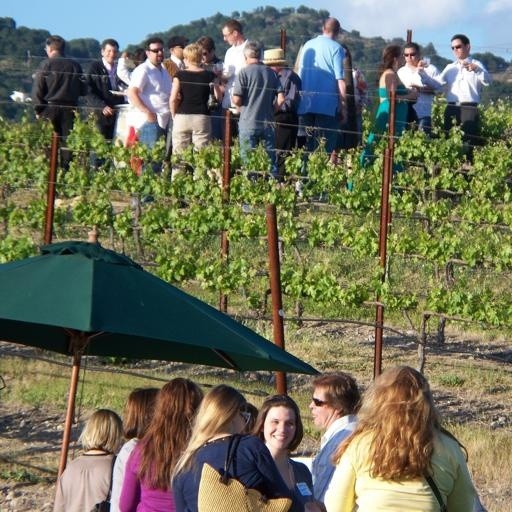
[262,48,287,64]
[168,36,190,47]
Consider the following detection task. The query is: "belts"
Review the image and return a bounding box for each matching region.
[448,102,477,106]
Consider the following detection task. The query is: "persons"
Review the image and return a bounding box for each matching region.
[81,38,123,193]
[396,42,445,136]
[232,42,286,185]
[348,45,419,193]
[53,367,488,512]
[32,35,85,205]
[258,48,302,184]
[218,20,250,145]
[290,18,367,200]
[416,34,492,142]
[116,35,224,202]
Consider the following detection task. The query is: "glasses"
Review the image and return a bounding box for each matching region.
[148,47,164,53]
[311,396,330,408]
[403,52,416,57]
[452,45,462,50]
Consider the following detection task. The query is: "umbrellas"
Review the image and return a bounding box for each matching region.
[0,224,323,487]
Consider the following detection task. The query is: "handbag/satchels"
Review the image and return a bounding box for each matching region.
[197,463,293,512]
